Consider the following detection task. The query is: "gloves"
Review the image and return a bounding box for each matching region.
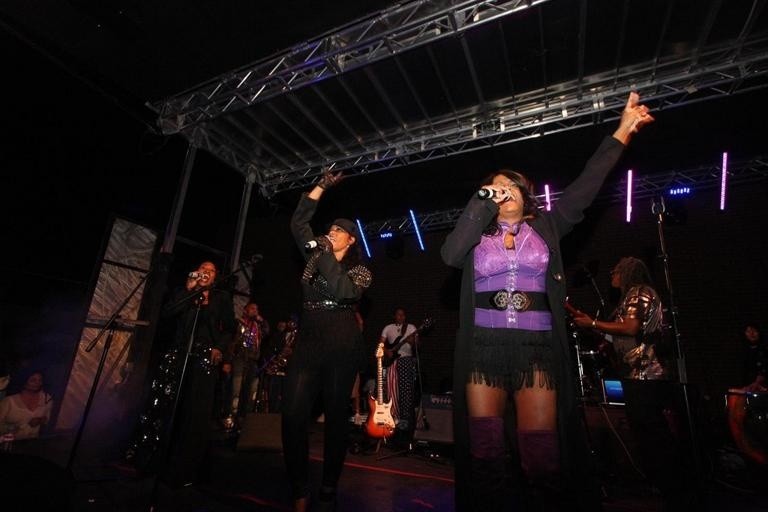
[315,234,334,255]
[318,167,345,190]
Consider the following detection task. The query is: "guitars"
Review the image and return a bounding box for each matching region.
[564,296,613,344]
[363,341,396,438]
[382,317,437,367]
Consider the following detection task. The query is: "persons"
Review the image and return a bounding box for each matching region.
[277,167,377,508]
[574,256,671,491]
[1,304,768,511]
[160,259,235,486]
[437,92,656,511]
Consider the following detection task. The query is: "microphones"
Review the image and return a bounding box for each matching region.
[304,234,336,251]
[475,188,510,204]
[187,270,205,280]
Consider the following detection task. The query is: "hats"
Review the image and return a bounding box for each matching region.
[330,218,360,238]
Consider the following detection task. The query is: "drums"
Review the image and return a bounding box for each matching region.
[727,388,768,466]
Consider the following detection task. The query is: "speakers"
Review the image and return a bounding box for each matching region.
[235,412,283,454]
[412,407,457,444]
